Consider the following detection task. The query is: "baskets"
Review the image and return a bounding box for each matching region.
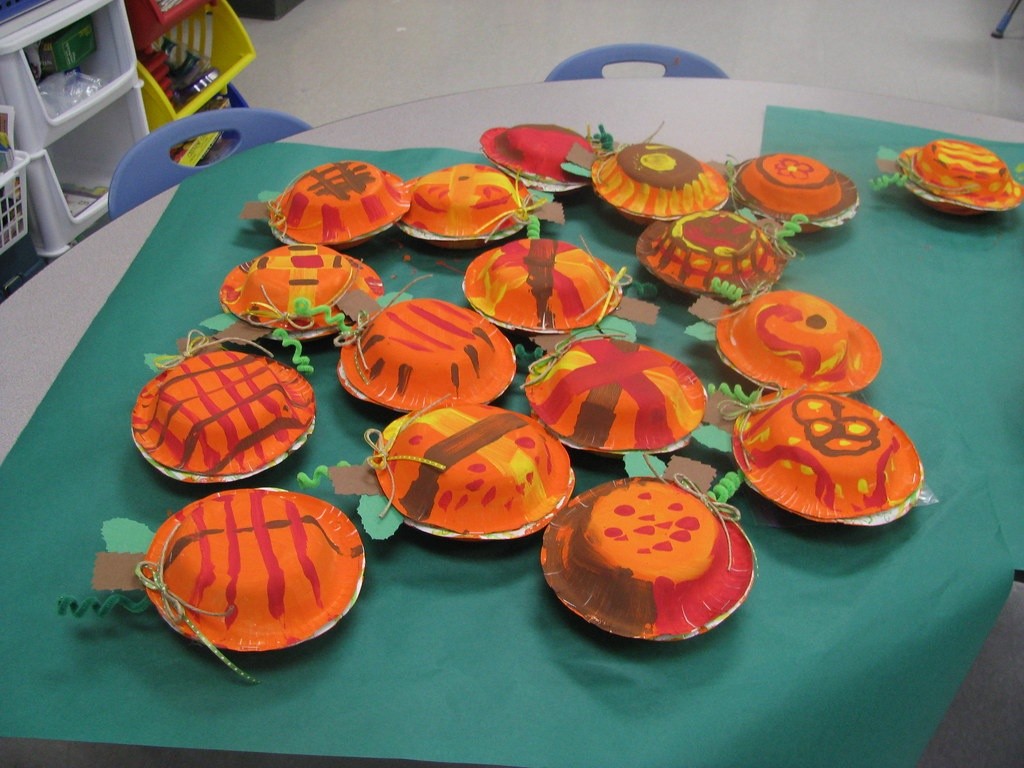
[0,150,31,255]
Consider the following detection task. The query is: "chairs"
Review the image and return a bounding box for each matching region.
[108,108,314,221]
[543,43,730,80]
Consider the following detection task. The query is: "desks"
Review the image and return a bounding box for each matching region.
[0,76,1024,768]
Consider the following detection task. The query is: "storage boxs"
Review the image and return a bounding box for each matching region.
[0,0,259,260]
